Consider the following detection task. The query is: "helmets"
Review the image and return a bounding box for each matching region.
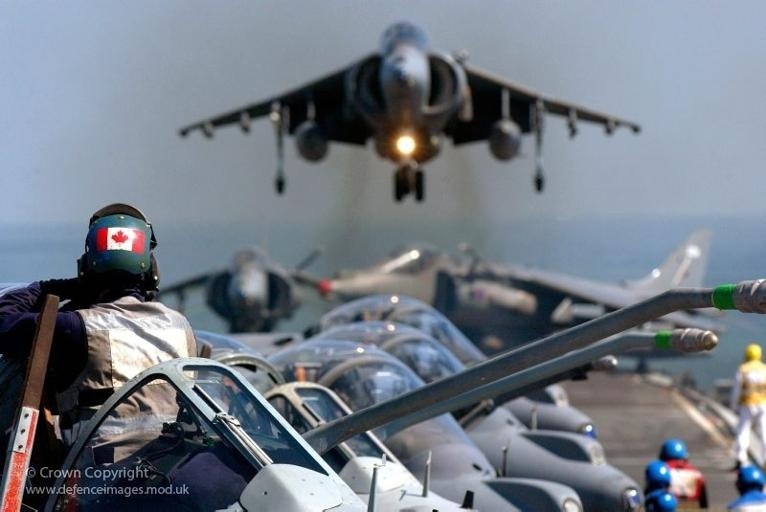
[77,203,159,293]
[745,344,762,360]
[737,465,765,485]
[644,440,686,512]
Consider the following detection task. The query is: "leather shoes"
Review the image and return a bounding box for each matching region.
[730,461,742,471]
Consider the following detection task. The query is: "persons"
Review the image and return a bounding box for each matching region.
[723,462,765,512]
[642,489,684,512]
[728,342,765,475]
[0,201,194,512]
[657,437,709,512]
[642,459,672,498]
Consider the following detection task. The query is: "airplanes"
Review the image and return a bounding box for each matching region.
[180,13,642,204]
